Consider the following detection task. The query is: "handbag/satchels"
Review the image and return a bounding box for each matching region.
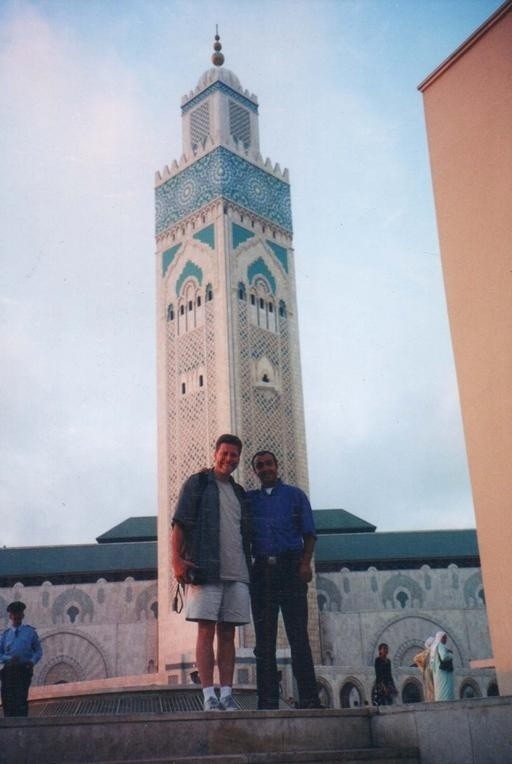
[438,661,454,672]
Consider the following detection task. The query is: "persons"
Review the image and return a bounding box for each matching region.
[414,636,435,702]
[198,450,329,710]
[171,434,253,711]
[0,602,43,717]
[429,630,455,702]
[370,643,399,706]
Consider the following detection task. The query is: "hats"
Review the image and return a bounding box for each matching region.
[7,601,26,612]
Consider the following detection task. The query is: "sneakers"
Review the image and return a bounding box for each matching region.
[203,695,238,712]
